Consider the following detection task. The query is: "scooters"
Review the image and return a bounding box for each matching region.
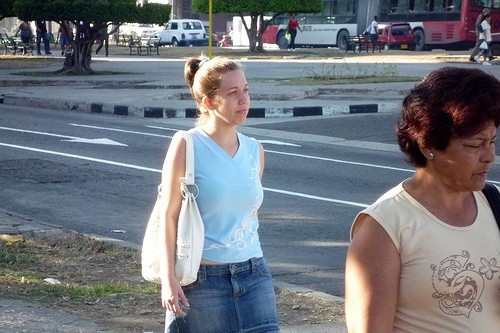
[218,32,233,47]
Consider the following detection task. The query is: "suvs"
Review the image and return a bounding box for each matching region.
[352,22,417,51]
[150,19,206,48]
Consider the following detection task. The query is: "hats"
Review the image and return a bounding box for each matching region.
[229,27,233,30]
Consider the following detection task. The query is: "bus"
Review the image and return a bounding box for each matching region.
[260,0,500,50]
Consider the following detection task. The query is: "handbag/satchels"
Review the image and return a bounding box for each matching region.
[142,130,205,286]
[285,33,290,40]
[286,27,290,31]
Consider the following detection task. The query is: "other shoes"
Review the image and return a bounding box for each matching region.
[45,52,52,54]
[475,56,479,63]
[37,52,41,55]
[61,52,64,56]
[288,49,291,51]
[483,61,492,65]
[293,49,295,51]
[488,56,497,61]
[96,50,98,56]
[106,55,110,57]
[469,56,477,62]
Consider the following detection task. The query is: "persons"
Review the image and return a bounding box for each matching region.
[286,13,303,52]
[158,55,281,333]
[95,22,111,58]
[35,19,53,55]
[58,20,74,56]
[14,20,34,55]
[473,11,492,67]
[343,66,500,333]
[370,15,380,54]
[469,8,498,62]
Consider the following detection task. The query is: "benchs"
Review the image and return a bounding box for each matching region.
[128,37,162,56]
[0,33,35,56]
[344,33,383,54]
[114,34,134,47]
